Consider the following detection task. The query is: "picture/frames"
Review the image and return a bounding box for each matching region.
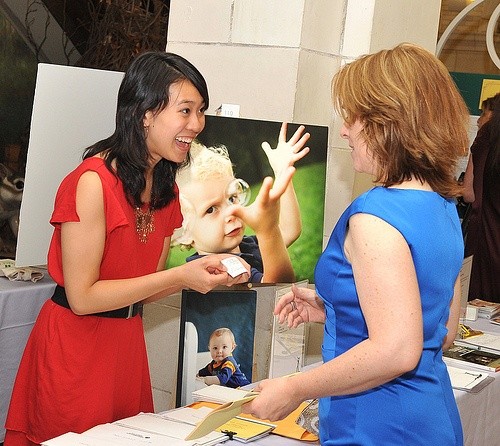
[175,290,257,407]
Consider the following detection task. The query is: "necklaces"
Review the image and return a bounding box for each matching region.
[135,206,156,243]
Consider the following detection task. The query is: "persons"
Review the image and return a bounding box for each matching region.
[196,328,250,389]
[462,93,500,304]
[3,51,251,446]
[242,43,469,446]
[170,121,310,284]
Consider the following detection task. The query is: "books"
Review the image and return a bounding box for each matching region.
[442,299,500,395]
[39,384,277,446]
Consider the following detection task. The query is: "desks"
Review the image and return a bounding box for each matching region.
[40,301,500,446]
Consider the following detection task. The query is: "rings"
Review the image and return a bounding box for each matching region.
[289,301,295,310]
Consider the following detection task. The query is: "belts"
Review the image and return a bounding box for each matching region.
[50,285,143,320]
